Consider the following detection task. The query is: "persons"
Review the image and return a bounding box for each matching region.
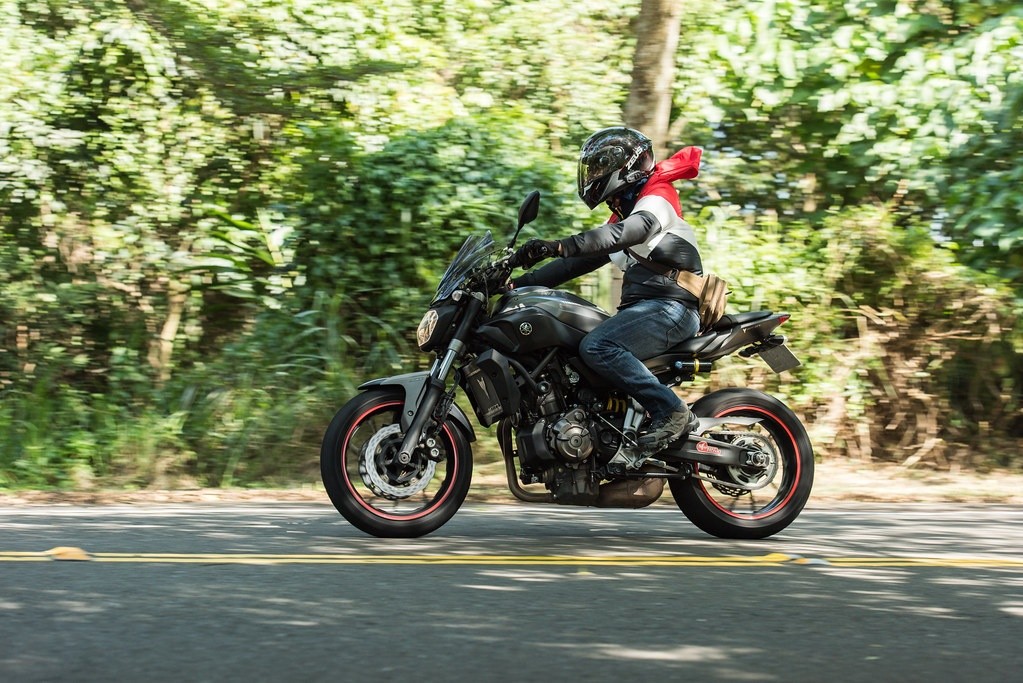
[509,128,704,450]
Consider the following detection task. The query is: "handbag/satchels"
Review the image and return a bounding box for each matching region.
[697,273,728,331]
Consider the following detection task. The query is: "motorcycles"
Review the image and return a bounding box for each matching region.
[318,190,817,540]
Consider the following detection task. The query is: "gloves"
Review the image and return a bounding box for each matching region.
[508,272,538,287]
[516,239,559,270]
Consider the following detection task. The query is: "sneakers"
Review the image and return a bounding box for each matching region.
[638,399,700,452]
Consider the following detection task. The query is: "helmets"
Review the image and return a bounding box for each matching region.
[578,127,655,209]
